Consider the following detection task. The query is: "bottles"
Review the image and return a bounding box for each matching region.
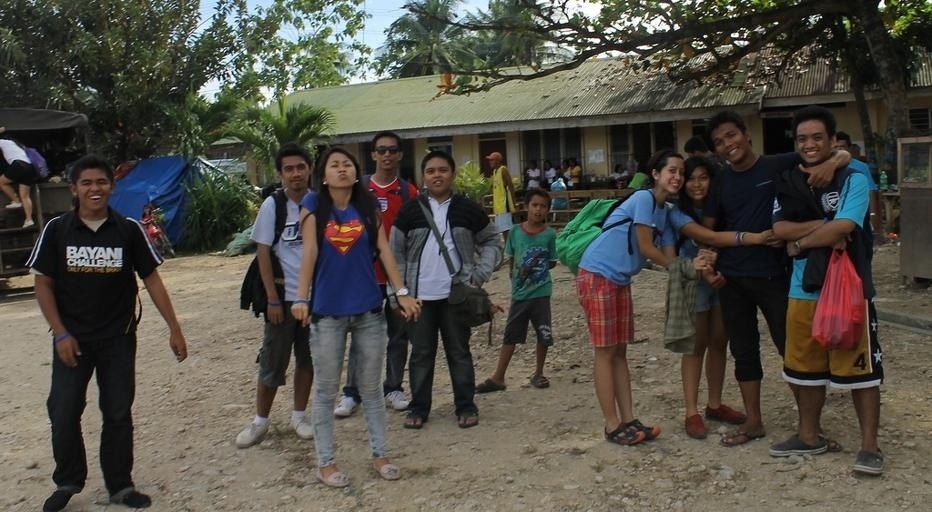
[879,171,889,193]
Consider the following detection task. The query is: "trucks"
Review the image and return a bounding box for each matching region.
[0,103,97,301]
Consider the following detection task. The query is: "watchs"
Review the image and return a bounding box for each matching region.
[395,286,409,297]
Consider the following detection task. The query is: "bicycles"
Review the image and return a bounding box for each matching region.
[139,206,176,258]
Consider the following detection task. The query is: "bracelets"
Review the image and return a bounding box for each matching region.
[292,299,310,305]
[54,331,69,345]
[267,300,282,307]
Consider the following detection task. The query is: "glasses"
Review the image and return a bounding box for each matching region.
[373,146,400,155]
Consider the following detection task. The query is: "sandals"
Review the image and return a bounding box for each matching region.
[372,457,403,481]
[316,463,350,487]
[604,423,646,445]
[622,418,662,441]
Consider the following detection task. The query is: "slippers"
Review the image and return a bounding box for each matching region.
[403,408,426,429]
[719,429,766,447]
[455,407,479,428]
[530,374,549,388]
[823,436,843,452]
[474,378,506,393]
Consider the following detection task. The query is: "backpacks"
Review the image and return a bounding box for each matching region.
[551,188,656,280]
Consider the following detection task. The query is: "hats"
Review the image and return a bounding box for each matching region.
[486,152,502,162]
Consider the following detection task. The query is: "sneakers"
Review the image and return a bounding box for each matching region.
[853,447,886,476]
[768,432,827,456]
[234,417,271,449]
[43,487,78,512]
[684,413,707,439]
[290,416,314,440]
[384,390,413,411]
[704,403,748,424]
[332,394,359,416]
[3,201,23,209]
[121,489,153,508]
[22,218,35,228]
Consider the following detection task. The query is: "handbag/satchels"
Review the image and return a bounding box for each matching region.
[444,281,500,336]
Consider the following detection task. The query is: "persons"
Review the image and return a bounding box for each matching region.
[289,143,426,491]
[485,151,519,246]
[23,152,188,512]
[232,142,319,451]
[0,135,35,229]
[470,187,560,394]
[384,149,505,429]
[330,129,424,419]
[139,205,165,246]
